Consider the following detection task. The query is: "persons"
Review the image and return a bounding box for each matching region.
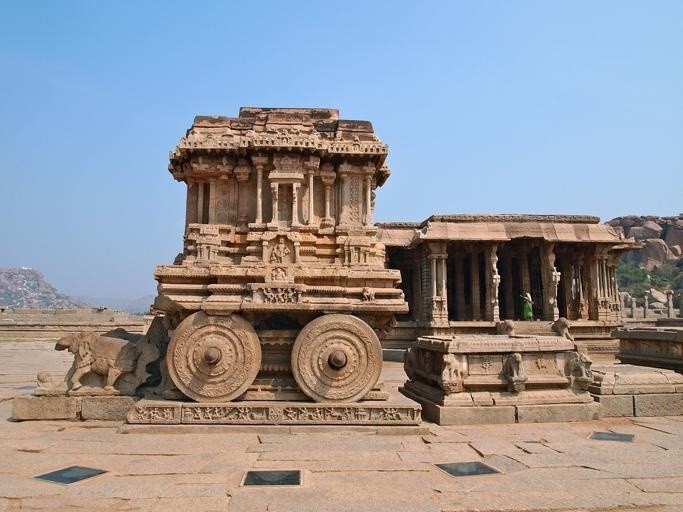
[521,290,533,320]
